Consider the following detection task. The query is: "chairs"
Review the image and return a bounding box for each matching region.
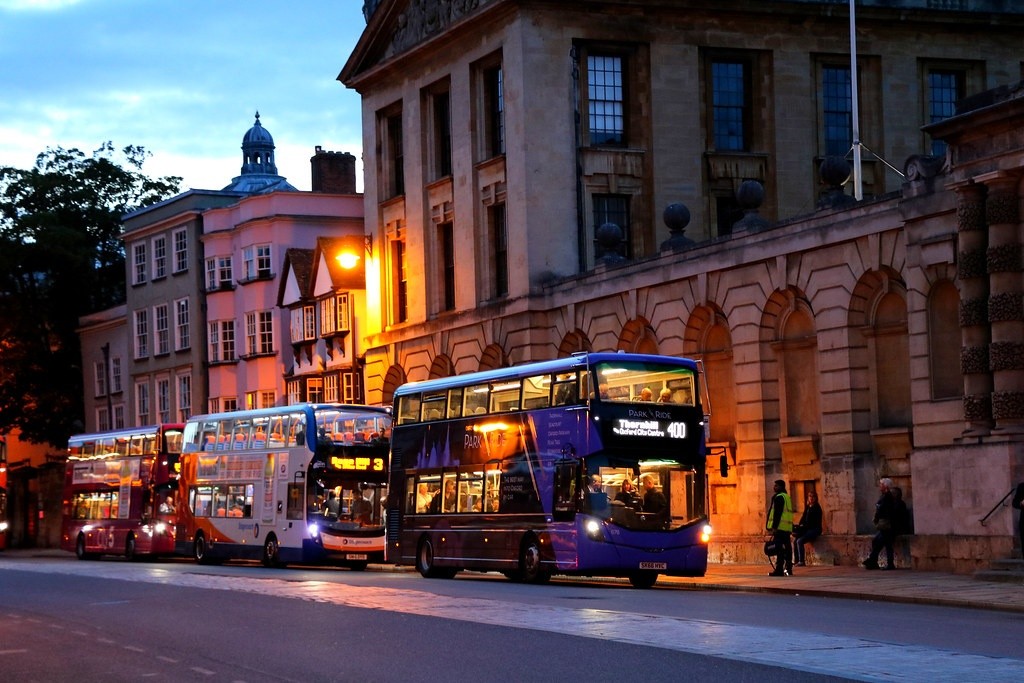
[428,408,441,420]
[449,405,486,417]
[201,433,380,451]
[216,507,243,517]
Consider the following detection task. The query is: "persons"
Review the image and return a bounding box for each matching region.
[581,474,601,500]
[641,388,652,401]
[863,478,913,570]
[351,491,372,523]
[310,495,323,511]
[590,383,609,399]
[614,479,643,511]
[582,367,608,400]
[159,496,175,513]
[556,373,584,405]
[766,479,793,576]
[205,493,221,516]
[659,388,672,403]
[322,492,344,516]
[416,480,499,513]
[376,428,389,443]
[1012,482,1024,559]
[641,475,666,530]
[318,428,332,444]
[792,491,822,567]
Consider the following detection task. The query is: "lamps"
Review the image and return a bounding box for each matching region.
[335,232,373,269]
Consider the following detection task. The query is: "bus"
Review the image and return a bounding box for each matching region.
[385,350,729,589]
[175,402,393,570]
[58,422,188,561]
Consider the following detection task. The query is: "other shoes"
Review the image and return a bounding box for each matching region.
[794,561,806,567]
[865,563,879,570]
[885,564,895,570]
[768,568,784,576]
[792,561,800,565]
[784,569,792,576]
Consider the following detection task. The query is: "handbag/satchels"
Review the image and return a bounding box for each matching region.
[764,533,786,557]
